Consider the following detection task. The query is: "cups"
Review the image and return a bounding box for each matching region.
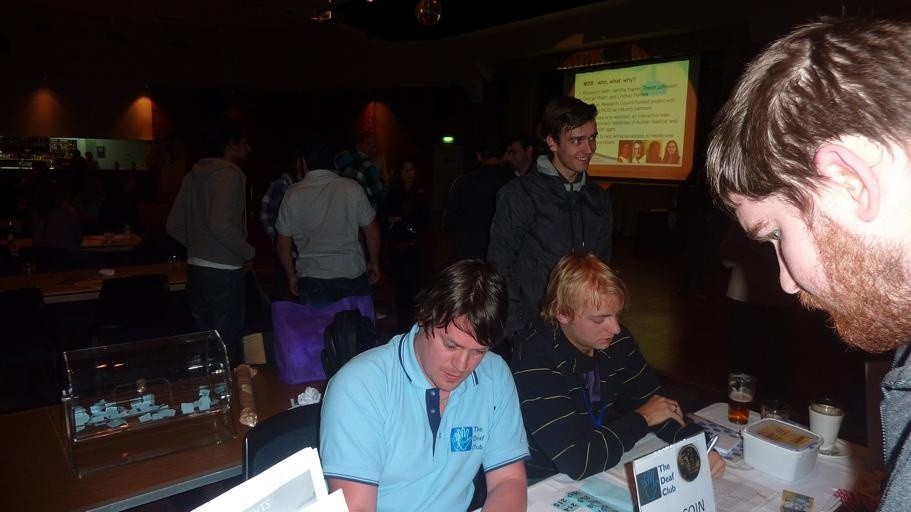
[808,403,846,451]
[123,224,132,237]
[167,255,178,274]
[760,400,793,423]
[727,371,758,425]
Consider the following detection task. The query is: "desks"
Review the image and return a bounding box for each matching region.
[471,400,888,511]
[0,207,87,229]
[1,365,329,512]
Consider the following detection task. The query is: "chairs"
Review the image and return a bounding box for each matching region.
[243,403,321,483]
[0,216,192,404]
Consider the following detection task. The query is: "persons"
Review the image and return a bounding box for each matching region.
[646,141,662,163]
[14,150,139,230]
[487,92,617,352]
[662,140,681,165]
[632,140,646,164]
[163,118,542,384]
[618,140,632,163]
[319,254,531,512]
[703,14,911,510]
[510,248,727,488]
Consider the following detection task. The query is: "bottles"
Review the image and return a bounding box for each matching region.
[0,151,44,160]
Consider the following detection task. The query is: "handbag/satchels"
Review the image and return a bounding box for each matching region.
[271,296,382,384]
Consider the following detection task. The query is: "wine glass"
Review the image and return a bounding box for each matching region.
[49,160,55,170]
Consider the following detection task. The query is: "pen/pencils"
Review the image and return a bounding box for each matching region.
[706,435,720,454]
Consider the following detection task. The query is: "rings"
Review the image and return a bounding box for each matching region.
[672,404,678,412]
